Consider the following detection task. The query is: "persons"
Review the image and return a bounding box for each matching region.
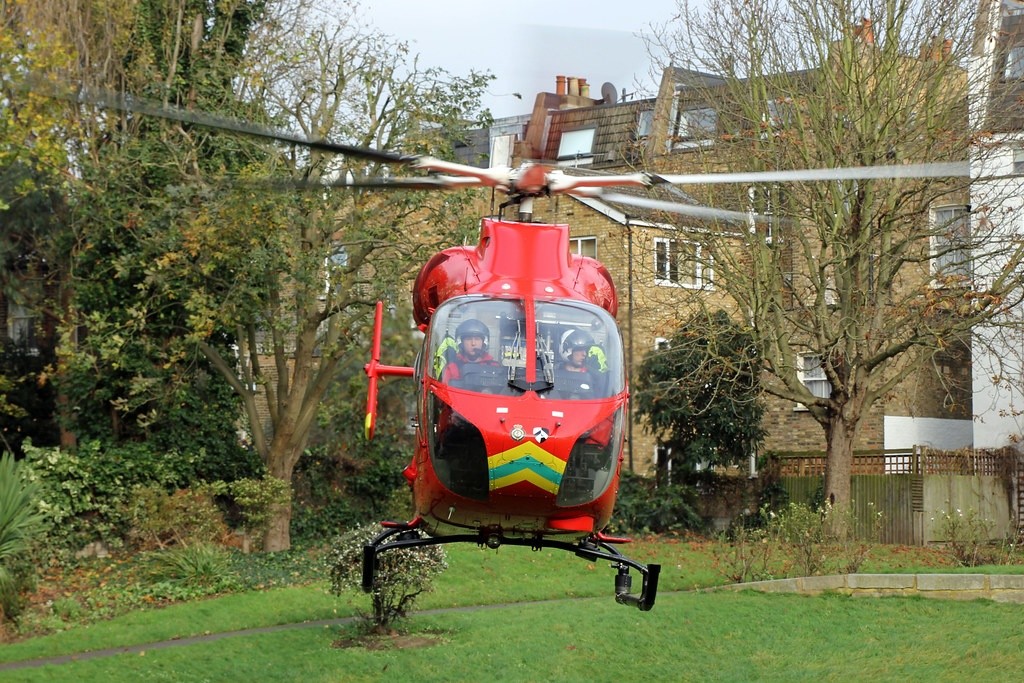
[554,330,600,373]
[438,320,507,442]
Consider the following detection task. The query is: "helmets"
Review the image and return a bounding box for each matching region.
[559,328,595,363]
[454,319,490,342]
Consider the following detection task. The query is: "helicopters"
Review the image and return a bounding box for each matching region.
[18,82,1023,622]
